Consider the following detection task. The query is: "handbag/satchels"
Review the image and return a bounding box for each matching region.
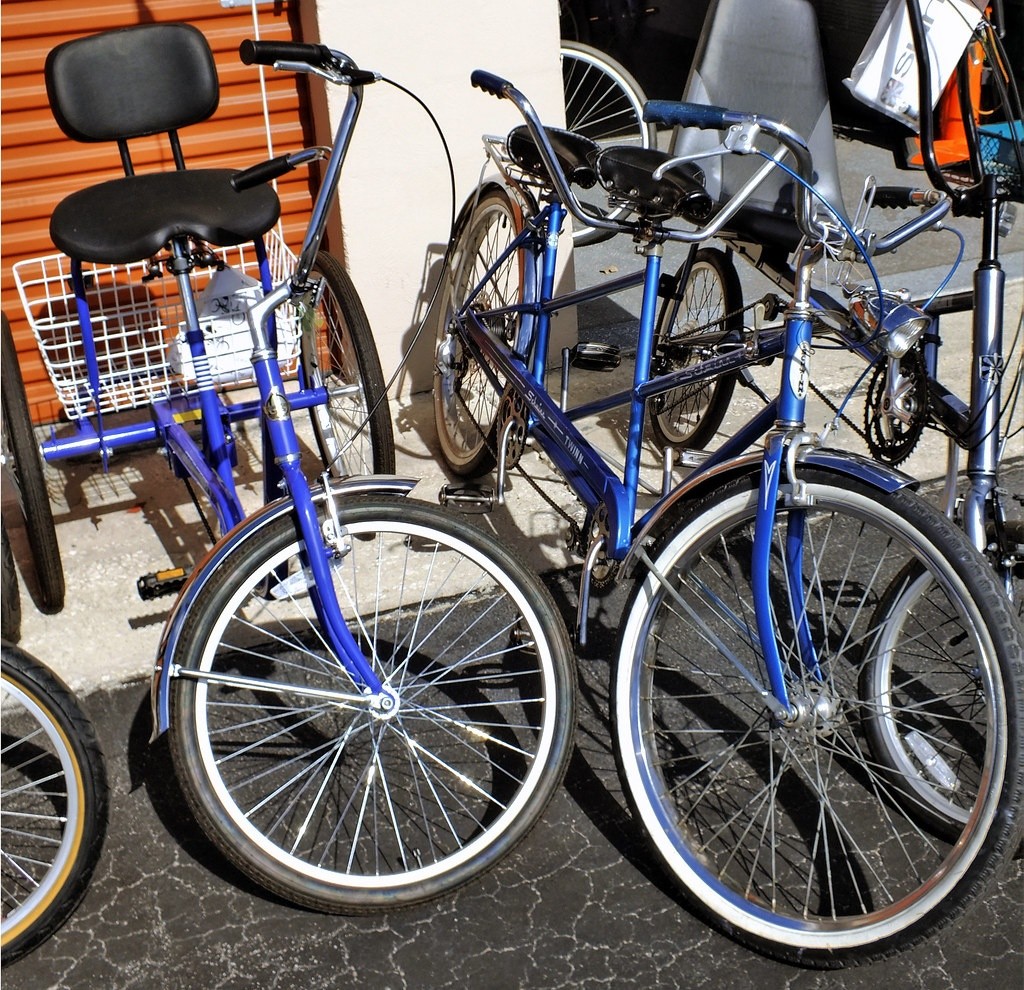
[843,0,983,134]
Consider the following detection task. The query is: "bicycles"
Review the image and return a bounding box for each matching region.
[426,37,1024,976]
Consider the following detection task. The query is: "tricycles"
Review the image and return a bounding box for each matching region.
[1,0,576,990]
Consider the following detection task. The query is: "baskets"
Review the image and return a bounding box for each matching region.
[14,227,310,420]
